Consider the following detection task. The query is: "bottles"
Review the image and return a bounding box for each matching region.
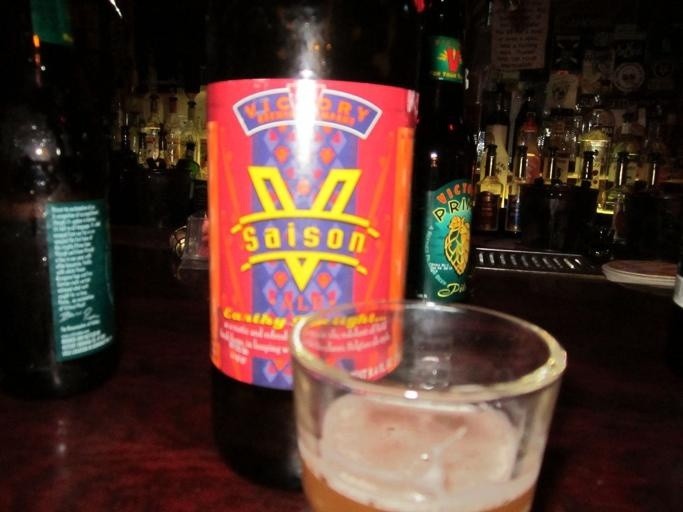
[199,3,427,492]
[1,2,124,402]
[119,67,208,218]
[482,68,681,244]
[417,3,481,309]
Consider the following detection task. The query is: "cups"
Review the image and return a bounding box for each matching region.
[291,296,566,511]
